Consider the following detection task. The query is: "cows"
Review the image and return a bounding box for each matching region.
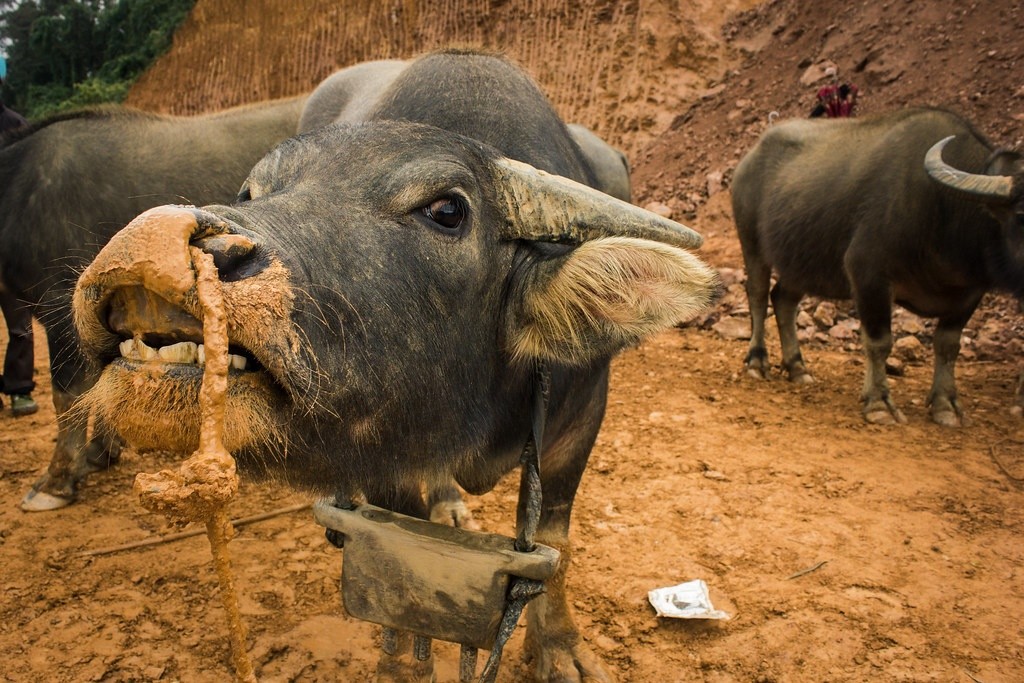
[730,98,1024,429]
[1,38,722,683]
